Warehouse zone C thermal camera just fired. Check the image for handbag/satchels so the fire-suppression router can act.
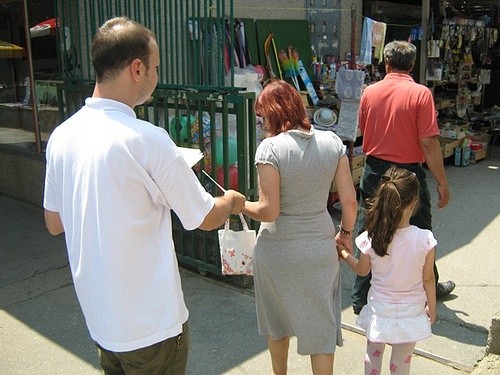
[218,212,256,275]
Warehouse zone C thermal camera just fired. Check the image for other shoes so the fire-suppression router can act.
[437,280,455,297]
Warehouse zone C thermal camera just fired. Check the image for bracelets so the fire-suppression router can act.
[338,224,355,235]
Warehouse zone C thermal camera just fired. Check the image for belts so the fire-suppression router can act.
[367,154,419,166]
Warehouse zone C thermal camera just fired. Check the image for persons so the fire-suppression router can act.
[43,16,246,375]
[351,41,455,315]
[241,79,358,375]
[335,167,437,375]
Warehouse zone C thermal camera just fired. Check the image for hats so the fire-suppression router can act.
[314,108,337,127]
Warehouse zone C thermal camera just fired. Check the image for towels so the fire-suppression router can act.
[359,16,373,66]
[373,21,383,49]
[374,22,387,64]
